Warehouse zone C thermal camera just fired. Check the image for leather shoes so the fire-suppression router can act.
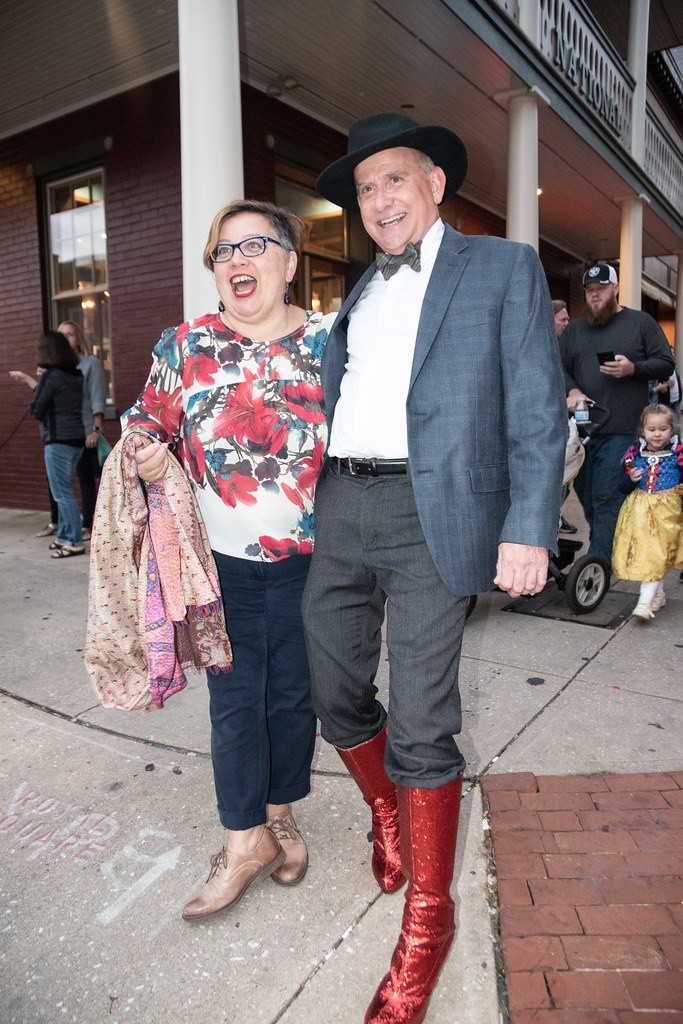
[266,806,309,887]
[181,825,286,922]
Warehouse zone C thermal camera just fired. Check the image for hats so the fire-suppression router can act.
[579,263,617,288]
[315,112,467,215]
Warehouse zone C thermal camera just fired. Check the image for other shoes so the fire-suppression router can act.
[36,525,57,537]
[82,532,90,541]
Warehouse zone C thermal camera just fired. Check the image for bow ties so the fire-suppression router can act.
[376,241,421,281]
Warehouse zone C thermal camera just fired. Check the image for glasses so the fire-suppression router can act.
[210,236,291,263]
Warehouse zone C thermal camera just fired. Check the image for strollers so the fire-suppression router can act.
[465,403,611,619]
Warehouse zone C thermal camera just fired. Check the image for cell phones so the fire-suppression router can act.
[596,350,616,365]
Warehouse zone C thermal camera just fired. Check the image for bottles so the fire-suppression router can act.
[575,399,589,421]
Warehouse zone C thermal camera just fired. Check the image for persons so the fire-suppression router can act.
[612,404,683,621]
[10,322,104,558]
[558,264,675,563]
[552,301,578,532]
[131,202,339,920]
[301,112,567,1024]
[649,345,683,411]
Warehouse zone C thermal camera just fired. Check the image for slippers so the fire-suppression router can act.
[52,548,84,558]
[49,543,61,549]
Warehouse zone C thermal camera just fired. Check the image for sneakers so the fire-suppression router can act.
[631,605,655,620]
[652,591,666,612]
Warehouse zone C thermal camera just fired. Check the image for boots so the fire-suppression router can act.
[334,725,407,895]
[364,773,463,1024]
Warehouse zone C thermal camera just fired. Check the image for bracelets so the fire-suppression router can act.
[93,426,101,431]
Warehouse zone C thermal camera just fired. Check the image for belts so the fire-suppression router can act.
[332,456,408,477]
